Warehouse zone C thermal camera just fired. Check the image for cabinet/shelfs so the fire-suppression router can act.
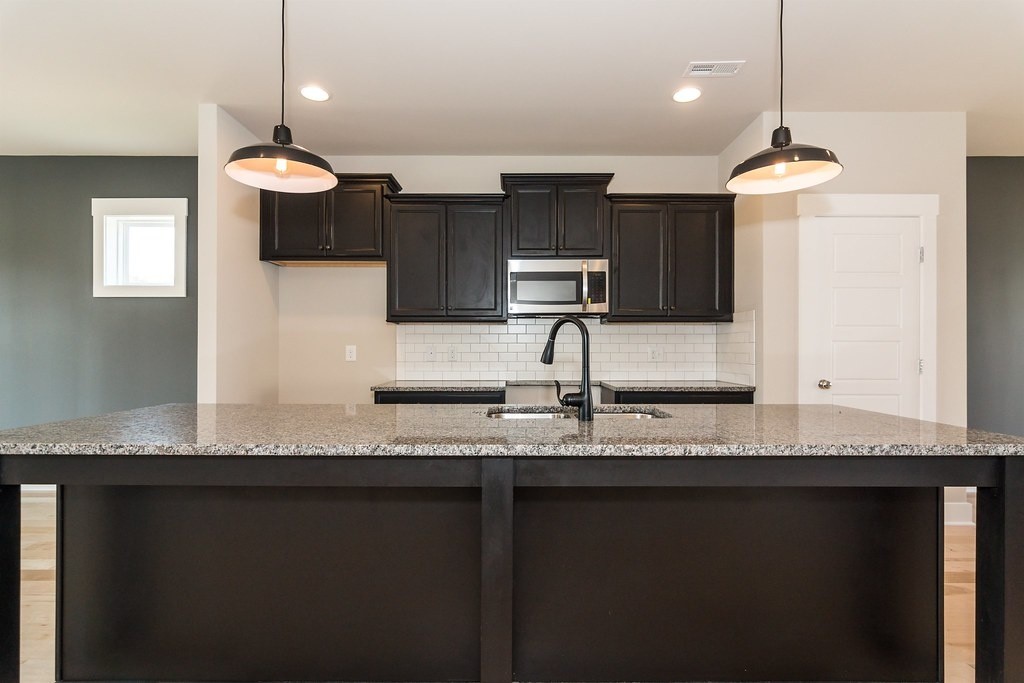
[606,192,737,323]
[500,170,614,258]
[260,171,405,268]
[385,192,508,324]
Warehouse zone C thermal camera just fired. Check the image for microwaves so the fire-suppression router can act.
[507,259,609,316]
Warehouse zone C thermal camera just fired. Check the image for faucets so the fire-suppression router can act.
[540,315,592,420]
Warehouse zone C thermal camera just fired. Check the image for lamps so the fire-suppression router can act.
[724,0,843,195]
[223,0,339,192]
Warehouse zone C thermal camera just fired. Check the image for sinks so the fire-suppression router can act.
[486,406,674,420]
